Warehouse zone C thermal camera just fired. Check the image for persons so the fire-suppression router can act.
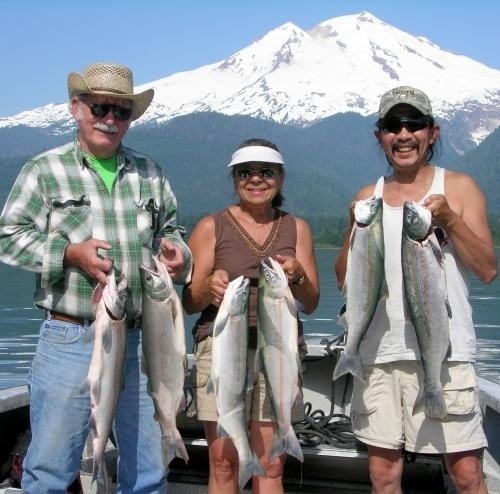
[333,85,497,494]
[182,138,321,493]
[0,62,193,494]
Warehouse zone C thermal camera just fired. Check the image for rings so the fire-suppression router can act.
[438,212,441,217]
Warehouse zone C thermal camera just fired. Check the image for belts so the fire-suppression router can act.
[50,308,144,331]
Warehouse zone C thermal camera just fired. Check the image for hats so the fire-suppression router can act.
[228,145,285,169]
[377,87,434,115]
[66,62,156,120]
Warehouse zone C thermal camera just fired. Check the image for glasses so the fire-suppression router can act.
[235,168,279,179]
[381,116,433,133]
[77,96,132,120]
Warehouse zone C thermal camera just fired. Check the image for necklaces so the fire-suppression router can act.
[245,211,270,237]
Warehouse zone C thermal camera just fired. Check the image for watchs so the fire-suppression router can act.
[292,273,307,288]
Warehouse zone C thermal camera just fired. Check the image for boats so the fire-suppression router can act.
[0,331,498,493]
